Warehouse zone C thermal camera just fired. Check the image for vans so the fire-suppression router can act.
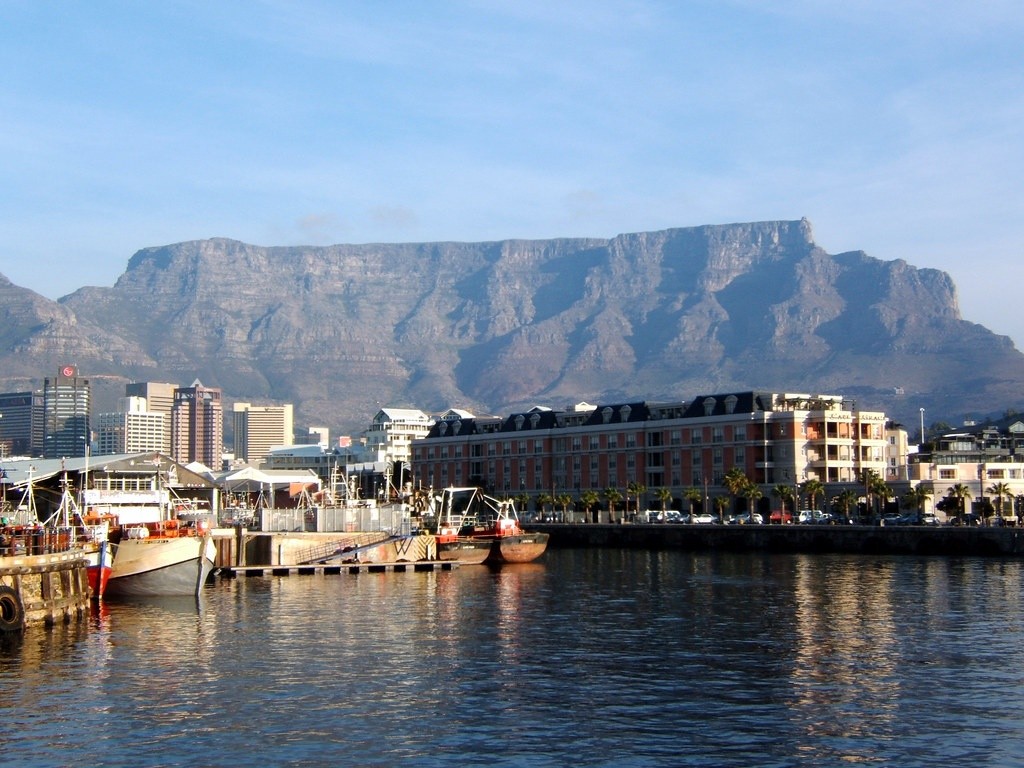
[649,511,680,523]
[792,510,822,524]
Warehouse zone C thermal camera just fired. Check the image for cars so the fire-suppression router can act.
[691,514,718,524]
[951,512,980,526]
[805,513,839,525]
[517,511,564,523]
[730,515,745,523]
[676,514,697,523]
[666,514,679,523]
[902,512,939,526]
[770,509,792,523]
[873,513,903,525]
[742,514,763,524]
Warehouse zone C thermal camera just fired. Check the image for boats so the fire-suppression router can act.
[437,483,494,565]
[44,457,113,601]
[494,494,550,563]
[108,463,218,597]
